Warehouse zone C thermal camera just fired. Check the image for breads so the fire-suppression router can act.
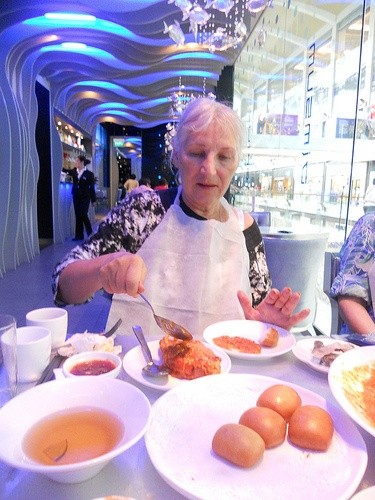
[260,326,278,346]
[238,406,286,449]
[256,384,301,422]
[159,335,221,379]
[288,404,336,450]
[212,423,265,468]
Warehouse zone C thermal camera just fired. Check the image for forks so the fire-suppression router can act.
[102,318,124,338]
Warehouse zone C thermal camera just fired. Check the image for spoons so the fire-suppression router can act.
[132,324,171,376]
[137,291,193,342]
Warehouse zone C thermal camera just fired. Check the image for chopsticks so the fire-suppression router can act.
[36,354,63,385]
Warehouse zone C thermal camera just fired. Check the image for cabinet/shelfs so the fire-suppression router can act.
[53,114,88,184]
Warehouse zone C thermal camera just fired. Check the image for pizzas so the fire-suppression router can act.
[211,336,261,354]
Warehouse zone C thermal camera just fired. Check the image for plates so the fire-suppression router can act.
[293,338,360,372]
[123,339,231,390]
[203,319,295,360]
[145,373,375,500]
[63,351,122,378]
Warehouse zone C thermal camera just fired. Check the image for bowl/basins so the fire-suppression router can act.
[0,375,151,485]
[328,345,375,437]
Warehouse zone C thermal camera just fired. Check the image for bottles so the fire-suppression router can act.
[59,131,85,161]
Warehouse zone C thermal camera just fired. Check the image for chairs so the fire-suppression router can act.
[262,233,329,335]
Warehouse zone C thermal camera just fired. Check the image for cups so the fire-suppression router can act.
[26,307,67,350]
[1,325,52,383]
[0,315,19,408]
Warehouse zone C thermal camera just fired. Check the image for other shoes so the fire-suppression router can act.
[71,237,85,242]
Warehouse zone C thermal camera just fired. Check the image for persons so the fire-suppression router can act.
[154,178,168,190]
[134,178,153,191]
[123,173,139,194]
[52,97,311,332]
[329,209,375,335]
[62,155,98,241]
[230,182,239,205]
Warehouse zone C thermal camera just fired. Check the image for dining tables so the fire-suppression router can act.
[0,332,375,500]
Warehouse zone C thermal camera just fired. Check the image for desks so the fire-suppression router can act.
[257,222,331,240]
